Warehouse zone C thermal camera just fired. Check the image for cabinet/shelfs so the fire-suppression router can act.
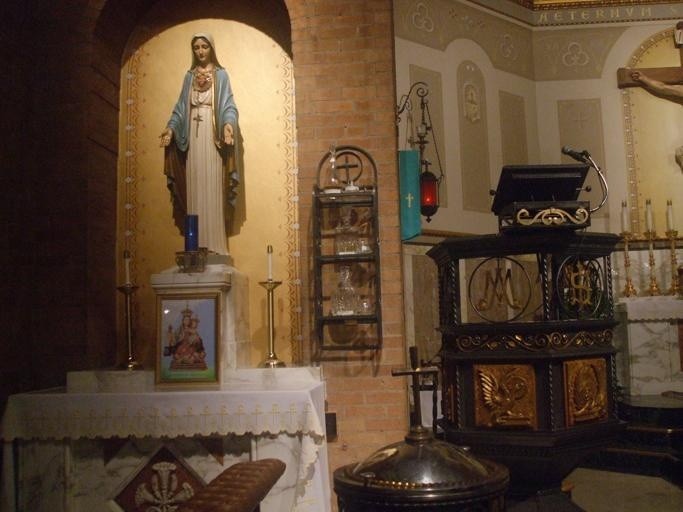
[313,145,383,351]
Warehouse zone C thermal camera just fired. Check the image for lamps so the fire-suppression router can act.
[395,82,446,223]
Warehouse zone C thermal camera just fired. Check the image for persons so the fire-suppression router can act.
[158,32,239,257]
[167,305,207,363]
[631,70,683,172]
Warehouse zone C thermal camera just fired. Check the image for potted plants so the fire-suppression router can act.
[622,201,629,232]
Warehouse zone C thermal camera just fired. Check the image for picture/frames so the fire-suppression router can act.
[154,290,221,390]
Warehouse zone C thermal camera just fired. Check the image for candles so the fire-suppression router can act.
[124,251,130,284]
[646,199,653,231]
[667,200,673,230]
[268,246,272,280]
